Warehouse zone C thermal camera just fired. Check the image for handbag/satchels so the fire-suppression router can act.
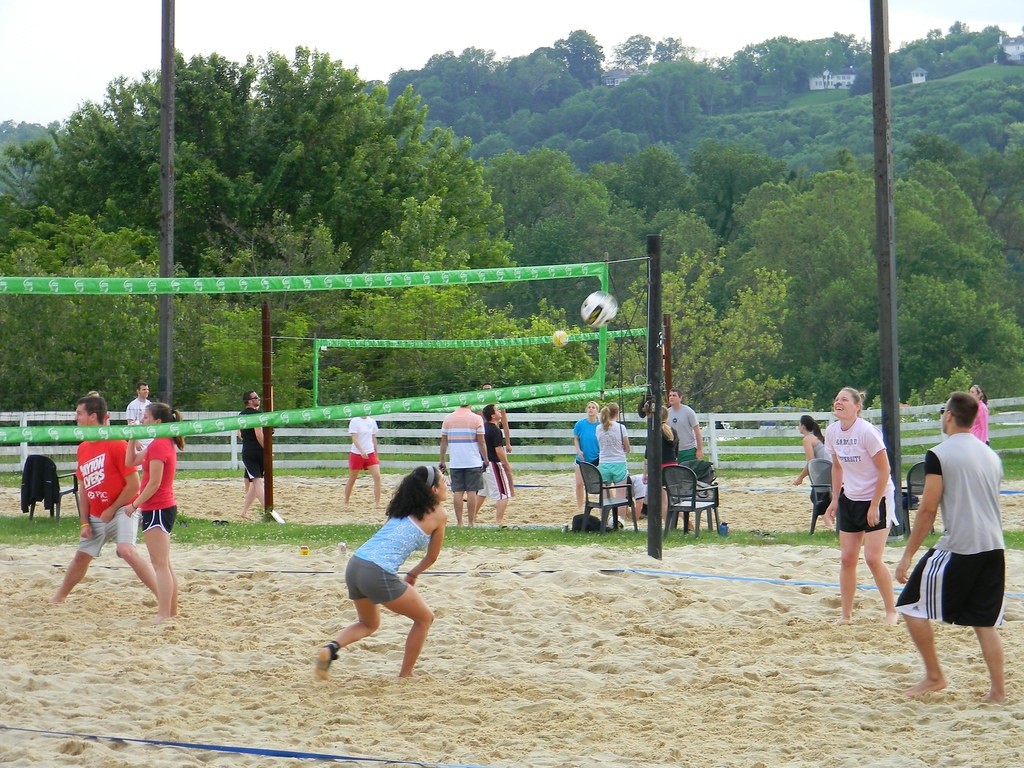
[679,459,717,499]
[572,514,600,532]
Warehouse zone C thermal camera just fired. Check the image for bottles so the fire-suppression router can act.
[337,541,347,554]
[301,546,309,555]
[720,522,728,536]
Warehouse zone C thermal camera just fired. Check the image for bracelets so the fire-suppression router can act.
[80,524,90,527]
[406,572,417,579]
[132,502,137,510]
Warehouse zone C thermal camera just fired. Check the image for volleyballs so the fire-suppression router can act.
[581,291,618,329]
[553,331,568,348]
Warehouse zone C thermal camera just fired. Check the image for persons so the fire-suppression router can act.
[236,389,274,519]
[644,404,695,532]
[343,399,387,509]
[626,473,649,523]
[792,415,844,531]
[474,404,512,525]
[469,384,515,497]
[316,465,448,682]
[573,401,602,518]
[124,402,185,619]
[664,386,704,465]
[971,385,988,446]
[438,405,489,529]
[46,390,159,606]
[824,387,898,627]
[596,402,631,523]
[893,392,1006,706]
[125,382,155,471]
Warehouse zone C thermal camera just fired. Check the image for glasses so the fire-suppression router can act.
[251,395,258,399]
[940,407,950,414]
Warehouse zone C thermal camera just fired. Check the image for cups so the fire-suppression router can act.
[561,524,568,532]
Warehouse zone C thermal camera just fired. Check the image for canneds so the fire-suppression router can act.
[338,542,346,554]
[562,523,568,533]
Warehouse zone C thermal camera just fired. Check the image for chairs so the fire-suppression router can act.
[901,462,935,534]
[807,458,844,534]
[662,464,720,538]
[21,455,81,523]
[576,460,638,536]
